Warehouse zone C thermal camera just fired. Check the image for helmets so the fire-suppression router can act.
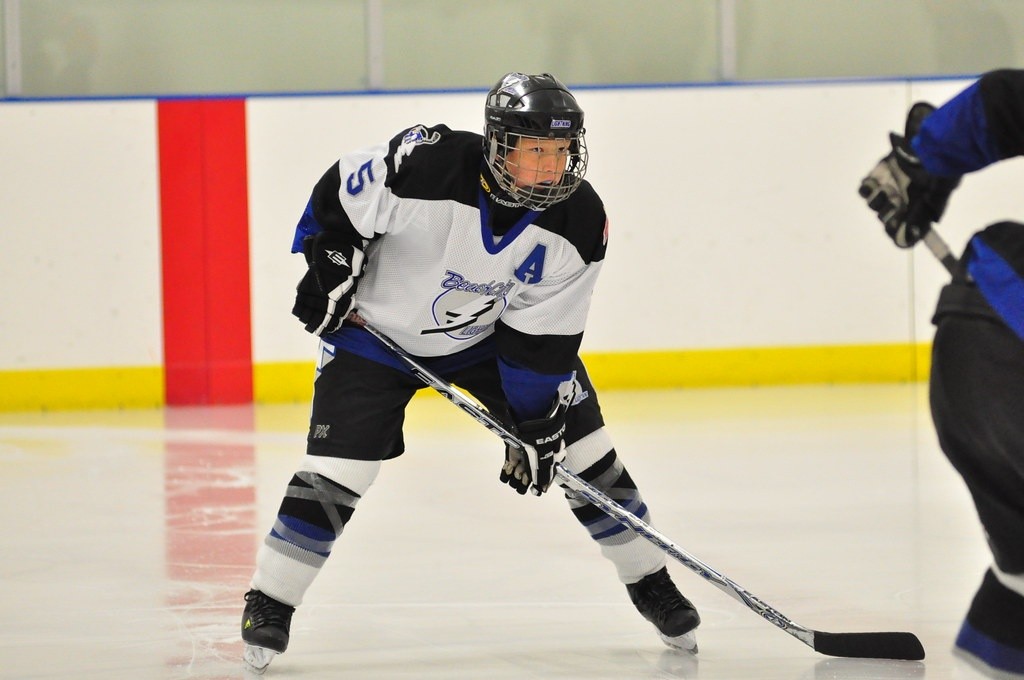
[484,71,585,209]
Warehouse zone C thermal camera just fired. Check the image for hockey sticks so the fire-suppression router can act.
[871,161,960,277]
[346,312,926,659]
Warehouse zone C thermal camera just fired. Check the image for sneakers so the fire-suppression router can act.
[625,566,701,655]
[240,588,296,674]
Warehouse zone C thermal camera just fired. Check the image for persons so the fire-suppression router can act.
[858,69,1024,679]
[241,73,702,672]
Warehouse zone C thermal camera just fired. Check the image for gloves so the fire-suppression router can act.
[859,102,961,249]
[291,230,369,336]
[499,385,566,497]
